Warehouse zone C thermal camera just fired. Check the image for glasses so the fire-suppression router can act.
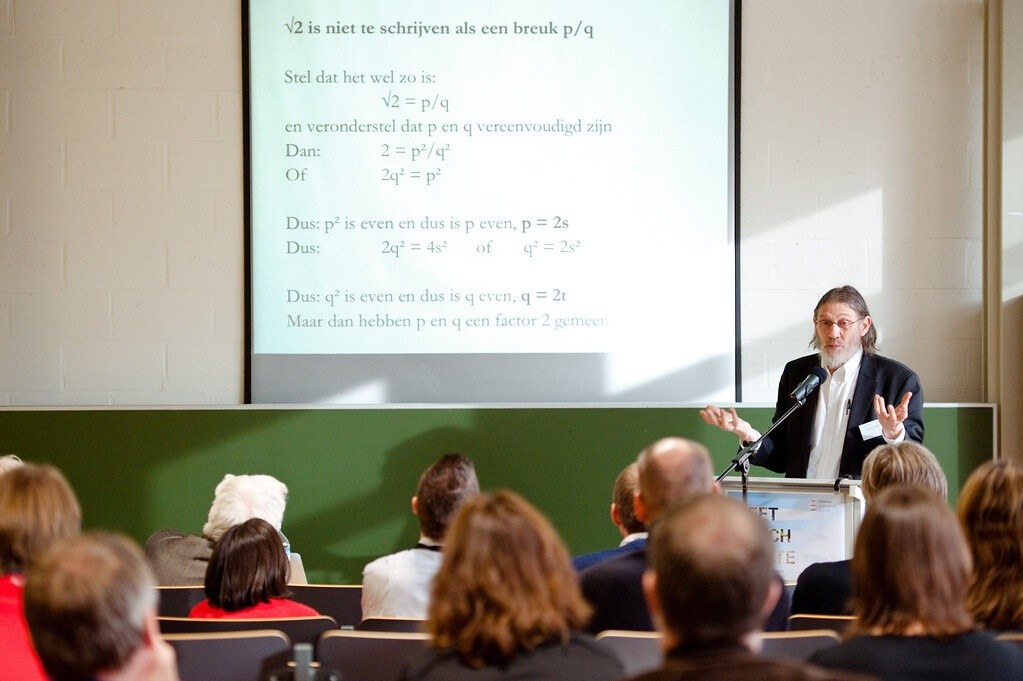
[813,317,865,329]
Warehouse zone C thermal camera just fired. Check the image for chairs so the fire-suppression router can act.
[152,587,859,680]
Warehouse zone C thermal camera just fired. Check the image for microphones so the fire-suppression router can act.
[788,367,827,403]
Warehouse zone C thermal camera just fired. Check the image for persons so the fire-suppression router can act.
[570,463,650,575]
[188,519,321,619]
[142,473,289,587]
[808,485,1023,681]
[580,436,789,638]
[0,464,80,681]
[360,454,480,620]
[958,462,1023,634]
[416,491,628,681]
[699,284,925,480]
[789,442,950,617]
[628,493,872,680]
[24,530,181,681]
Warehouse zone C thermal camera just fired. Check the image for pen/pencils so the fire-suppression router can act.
[846,399,852,415]
